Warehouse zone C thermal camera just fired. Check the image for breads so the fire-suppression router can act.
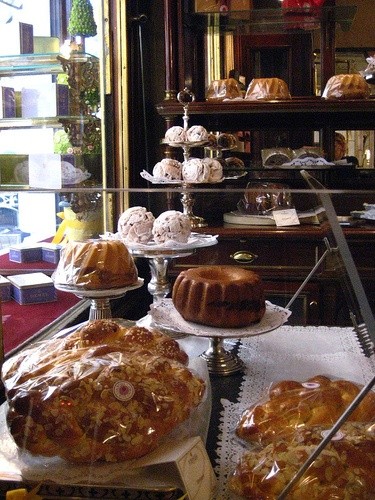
[152,124,223,182]
[172,265,265,329]
[56,240,138,290]
[225,374,375,500]
[205,133,245,177]
[1,319,206,465]
[116,206,192,246]
[332,133,345,160]
[208,77,291,100]
[240,182,292,216]
[321,74,370,100]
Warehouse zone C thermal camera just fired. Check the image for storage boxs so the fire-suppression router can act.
[9,241,63,265]
[0,272,56,306]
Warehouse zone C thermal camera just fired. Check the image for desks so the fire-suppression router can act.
[0,235,91,368]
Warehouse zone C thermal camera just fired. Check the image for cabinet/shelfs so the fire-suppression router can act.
[117,0,375,325]
[0,51,101,239]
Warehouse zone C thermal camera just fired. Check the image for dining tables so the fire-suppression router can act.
[0,318,375,500]
